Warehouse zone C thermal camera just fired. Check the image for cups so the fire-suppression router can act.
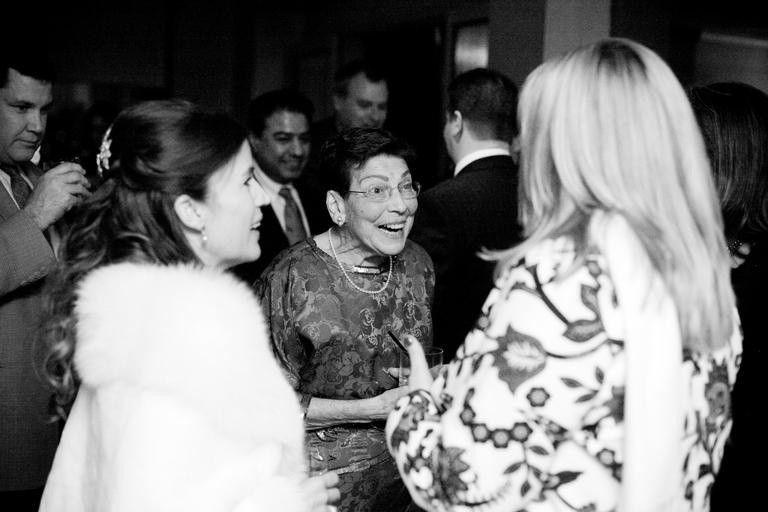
[43,155,83,200]
[399,346,443,387]
[307,445,330,476]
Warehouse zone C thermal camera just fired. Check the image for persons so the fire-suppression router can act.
[704,356,732,421]
[249,129,435,512]
[383,36,743,512]
[226,87,333,288]
[409,68,520,364]
[31,96,339,512]
[0,42,93,512]
[686,81,768,512]
[312,56,389,212]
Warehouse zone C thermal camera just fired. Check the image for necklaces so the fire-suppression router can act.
[327,225,396,298]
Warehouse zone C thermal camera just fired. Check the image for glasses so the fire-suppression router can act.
[340,179,422,203]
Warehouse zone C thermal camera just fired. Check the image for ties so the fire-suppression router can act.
[0,162,53,250]
[279,187,307,246]
